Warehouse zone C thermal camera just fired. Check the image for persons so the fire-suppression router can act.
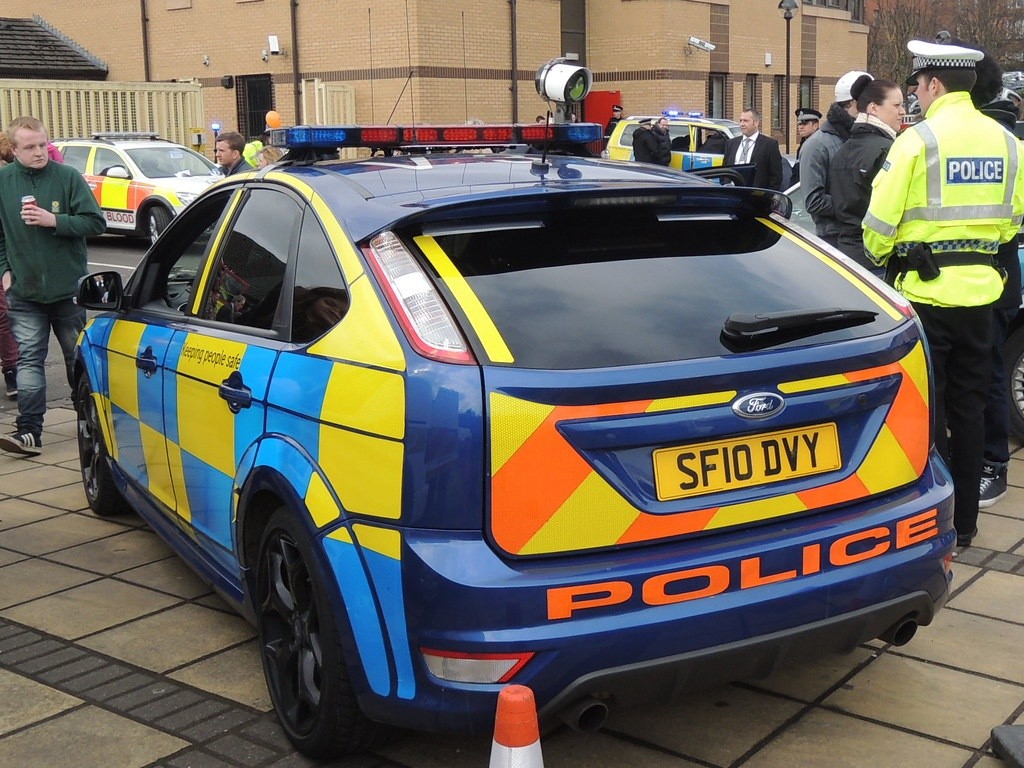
[530,115,550,155]
[720,109,783,193]
[944,41,1024,508]
[204,291,222,318]
[0,117,107,455]
[789,108,822,187]
[829,75,906,282]
[799,71,875,276]
[210,130,256,188]
[256,146,283,173]
[632,118,662,167]
[214,295,246,324]
[861,40,1024,559]
[572,113,579,123]
[650,118,671,169]
[603,104,626,153]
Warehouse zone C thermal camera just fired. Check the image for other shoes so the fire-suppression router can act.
[957,523,978,548]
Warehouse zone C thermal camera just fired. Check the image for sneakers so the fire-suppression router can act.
[0,422,42,455]
[978,465,1008,509]
[1,367,18,396]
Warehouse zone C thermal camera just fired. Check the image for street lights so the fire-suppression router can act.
[778,0,800,154]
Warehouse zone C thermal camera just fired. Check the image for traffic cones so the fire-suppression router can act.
[488,685,545,767]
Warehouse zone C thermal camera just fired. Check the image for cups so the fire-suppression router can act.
[21,196,37,223]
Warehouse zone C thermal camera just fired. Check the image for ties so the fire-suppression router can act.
[738,137,751,163]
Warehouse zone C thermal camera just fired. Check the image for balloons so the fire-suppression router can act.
[265,111,281,129]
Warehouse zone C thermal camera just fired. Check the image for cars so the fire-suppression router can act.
[605,110,741,187]
[73,122,960,768]
[904,70,1024,110]
[52,132,227,246]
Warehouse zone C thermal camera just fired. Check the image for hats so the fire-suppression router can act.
[639,118,652,125]
[794,108,822,121]
[612,105,623,112]
[834,70,875,102]
[897,39,985,85]
